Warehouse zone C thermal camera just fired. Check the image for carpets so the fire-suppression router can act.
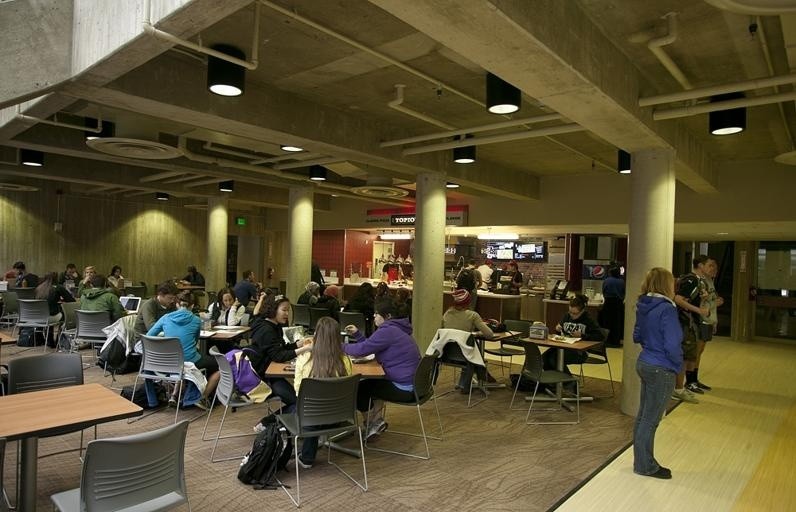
[0,328,691,510]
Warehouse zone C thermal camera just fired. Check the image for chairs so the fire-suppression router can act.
[267,373,368,507]
[201,346,284,463]
[575,328,614,399]
[363,348,446,460]
[3,352,98,509]
[509,338,581,425]
[50,420,190,510]
[0,273,535,427]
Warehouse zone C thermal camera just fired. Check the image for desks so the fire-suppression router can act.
[264,355,386,459]
[1,382,143,512]
[521,335,603,412]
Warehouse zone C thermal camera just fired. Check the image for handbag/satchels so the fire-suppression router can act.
[18,328,44,346]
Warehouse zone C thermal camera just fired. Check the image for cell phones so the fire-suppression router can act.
[284,366,296,370]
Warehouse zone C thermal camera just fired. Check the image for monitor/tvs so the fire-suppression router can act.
[486,241,514,261]
[513,241,545,261]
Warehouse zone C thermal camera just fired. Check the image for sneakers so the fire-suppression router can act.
[297,452,312,468]
[194,399,210,410]
[671,381,711,403]
[169,396,177,406]
[367,419,387,441]
[479,372,497,383]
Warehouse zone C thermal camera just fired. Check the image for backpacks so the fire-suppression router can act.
[456,268,475,290]
[225,348,272,404]
[238,425,292,490]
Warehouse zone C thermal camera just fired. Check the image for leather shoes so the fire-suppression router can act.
[650,467,671,478]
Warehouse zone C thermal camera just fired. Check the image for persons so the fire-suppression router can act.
[670,254,714,404]
[597,264,626,335]
[633,266,685,480]
[695,258,725,391]
[541,294,605,397]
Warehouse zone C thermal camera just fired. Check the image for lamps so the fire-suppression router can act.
[446,182,460,189]
[219,179,235,193]
[309,164,326,181]
[22,150,44,167]
[85,118,107,140]
[206,43,247,97]
[280,145,303,151]
[375,227,415,267]
[485,72,522,115]
[710,92,749,135]
[453,134,476,164]
[156,192,169,201]
[618,147,631,174]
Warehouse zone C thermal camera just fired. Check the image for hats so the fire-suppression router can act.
[452,289,472,305]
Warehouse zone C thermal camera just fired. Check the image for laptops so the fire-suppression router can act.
[119,297,142,314]
